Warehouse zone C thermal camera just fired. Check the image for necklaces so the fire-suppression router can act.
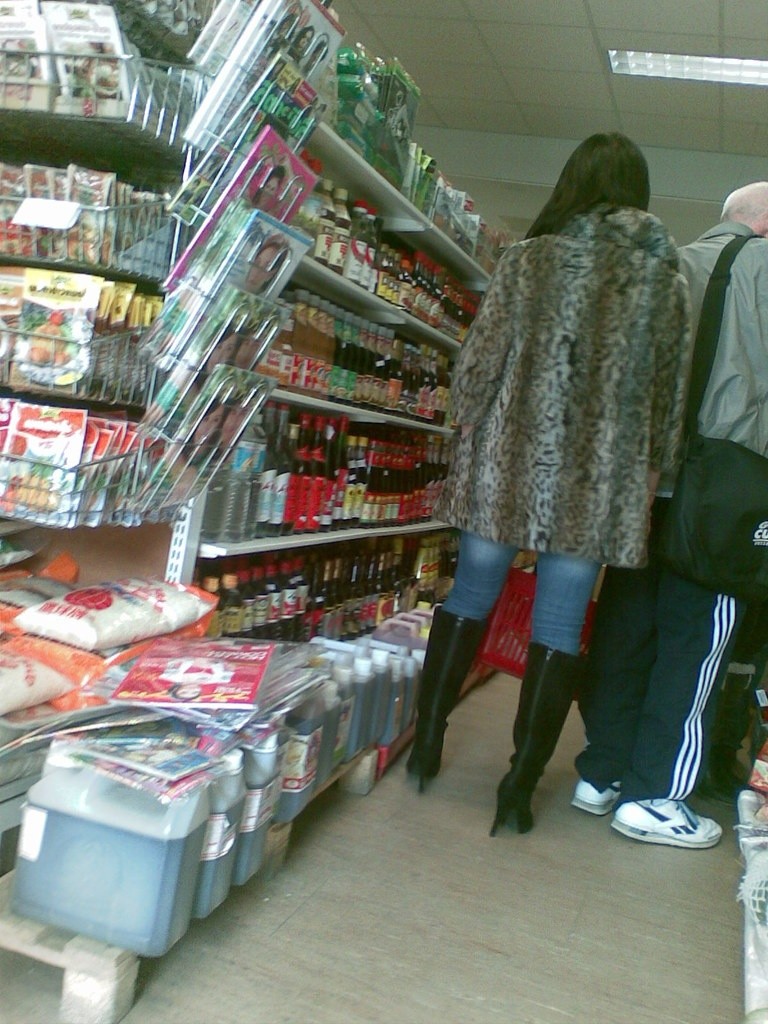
[252,166,286,214]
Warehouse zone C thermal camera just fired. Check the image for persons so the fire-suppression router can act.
[574,181,768,850]
[210,398,259,472]
[408,133,692,838]
[303,41,329,75]
[182,397,224,480]
[244,233,289,295]
[269,14,296,57]
[234,321,266,377]
[195,186,209,208]
[158,657,236,700]
[171,178,199,215]
[193,320,243,388]
[287,26,315,63]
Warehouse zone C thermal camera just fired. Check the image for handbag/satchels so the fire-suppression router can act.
[648,435,768,608]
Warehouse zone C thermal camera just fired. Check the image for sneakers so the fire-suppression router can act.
[571,778,622,816]
[610,799,723,849]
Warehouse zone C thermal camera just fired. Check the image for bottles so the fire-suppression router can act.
[293,150,480,345]
[199,402,449,541]
[12,602,442,958]
[194,533,463,644]
[259,283,456,428]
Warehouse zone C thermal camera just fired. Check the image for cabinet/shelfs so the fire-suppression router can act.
[0,0,492,835]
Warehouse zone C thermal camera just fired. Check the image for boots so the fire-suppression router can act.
[406,606,488,795]
[488,642,582,838]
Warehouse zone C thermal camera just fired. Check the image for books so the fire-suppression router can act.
[0,1,344,800]
